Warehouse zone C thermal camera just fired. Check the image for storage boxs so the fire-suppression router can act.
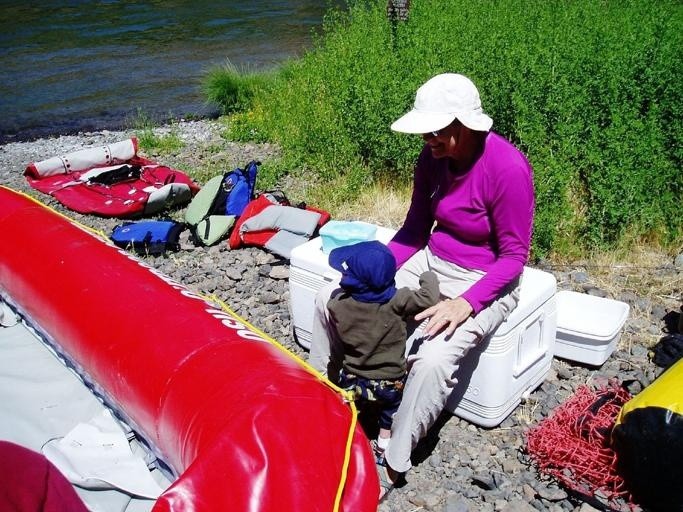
[556,292,627,367]
[291,224,556,427]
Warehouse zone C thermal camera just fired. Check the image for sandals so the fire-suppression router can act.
[375,457,405,504]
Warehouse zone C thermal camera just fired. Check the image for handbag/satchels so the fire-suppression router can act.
[208,160,261,216]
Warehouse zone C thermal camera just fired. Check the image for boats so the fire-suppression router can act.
[0,183,385,512]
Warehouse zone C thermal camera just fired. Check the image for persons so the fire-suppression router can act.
[322,238,440,467]
[303,69,536,505]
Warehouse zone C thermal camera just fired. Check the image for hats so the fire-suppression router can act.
[329,240,397,303]
[390,73,494,134]
